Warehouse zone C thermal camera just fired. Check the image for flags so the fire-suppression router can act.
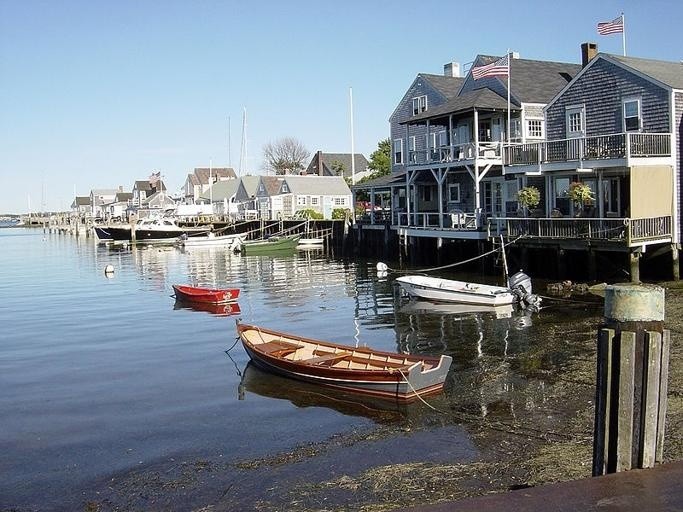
[472,57,510,78]
[596,15,624,34]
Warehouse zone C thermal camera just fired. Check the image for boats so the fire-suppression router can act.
[174,232,248,246]
[234,317,452,402]
[395,273,546,313]
[170,284,240,303]
[172,298,241,318]
[236,359,412,427]
[231,232,301,252]
[396,297,537,331]
[90,219,210,240]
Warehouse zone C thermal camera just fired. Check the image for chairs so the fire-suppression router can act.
[479,143,499,157]
[585,143,609,159]
[620,138,647,157]
[449,208,467,227]
